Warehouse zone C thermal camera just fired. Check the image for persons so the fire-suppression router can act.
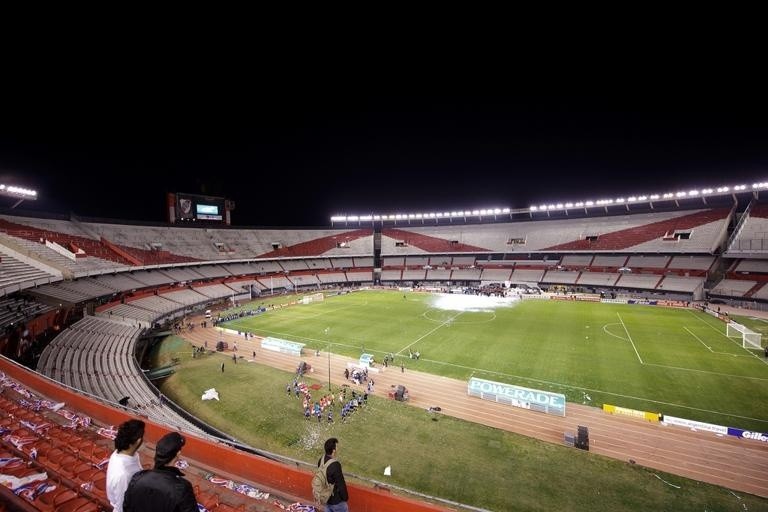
[316,436,350,511]
[285,366,376,426]
[105,417,146,512]
[399,359,405,373]
[120,431,202,512]
[172,306,264,372]
[381,351,395,369]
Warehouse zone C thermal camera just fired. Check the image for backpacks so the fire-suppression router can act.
[310,453,338,505]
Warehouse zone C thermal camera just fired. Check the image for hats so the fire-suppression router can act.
[154,431,186,458]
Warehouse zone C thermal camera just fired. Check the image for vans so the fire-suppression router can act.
[205,310,211,318]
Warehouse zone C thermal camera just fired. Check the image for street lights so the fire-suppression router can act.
[324,326,331,391]
